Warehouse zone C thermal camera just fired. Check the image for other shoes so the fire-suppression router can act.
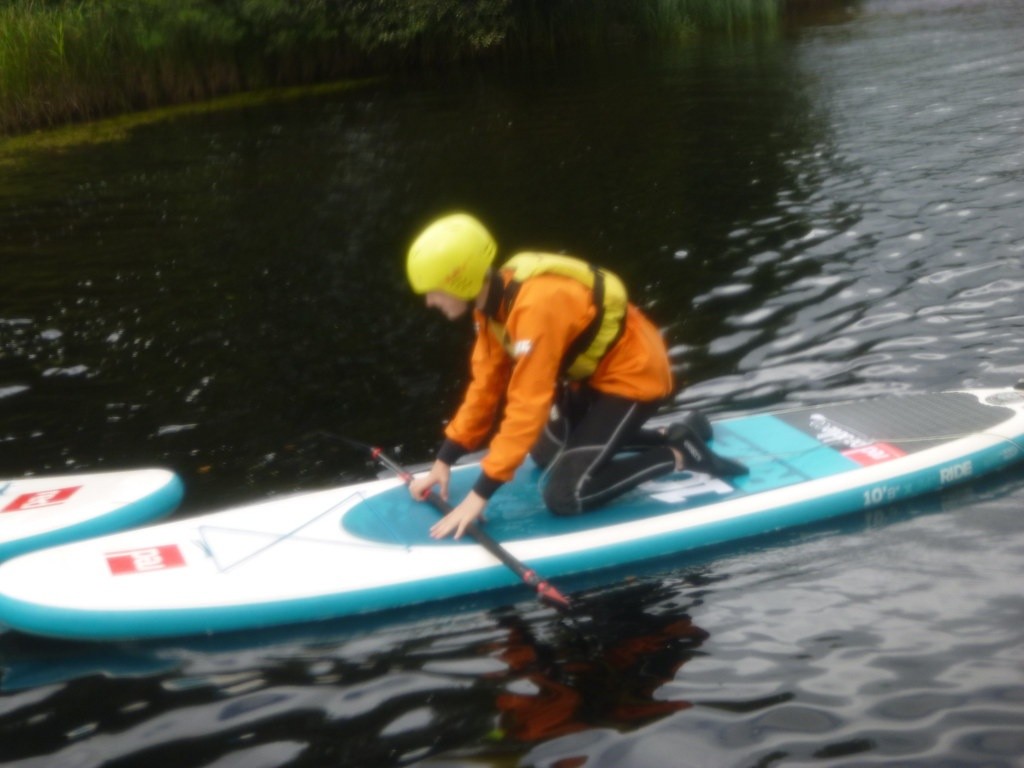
[665,422,748,476]
[684,408,713,440]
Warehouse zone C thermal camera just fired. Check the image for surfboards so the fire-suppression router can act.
[0,463,187,558]
[0,381,1022,646]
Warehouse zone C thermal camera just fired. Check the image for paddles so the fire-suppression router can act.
[364,440,579,613]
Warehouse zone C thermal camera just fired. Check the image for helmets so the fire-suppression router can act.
[406,212,497,300]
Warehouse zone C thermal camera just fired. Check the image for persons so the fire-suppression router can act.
[402,206,748,544]
